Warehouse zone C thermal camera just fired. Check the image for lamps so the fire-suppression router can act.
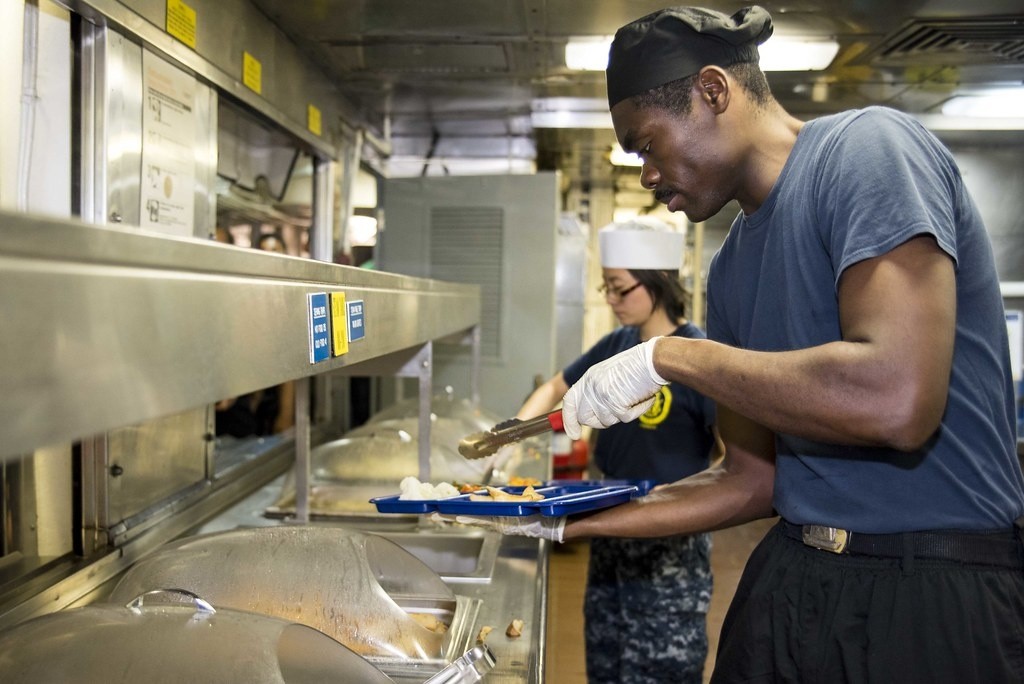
[562,33,843,71]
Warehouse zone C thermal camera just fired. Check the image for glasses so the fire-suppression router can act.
[598,281,643,301]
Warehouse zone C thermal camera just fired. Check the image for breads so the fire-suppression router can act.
[406,612,449,633]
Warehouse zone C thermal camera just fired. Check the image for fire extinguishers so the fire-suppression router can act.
[552,428,589,481]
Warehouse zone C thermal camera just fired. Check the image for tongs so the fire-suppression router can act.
[458,394,657,460]
[423,644,495,684]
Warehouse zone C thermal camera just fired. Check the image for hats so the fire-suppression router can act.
[599,217,683,269]
[606,5,773,111]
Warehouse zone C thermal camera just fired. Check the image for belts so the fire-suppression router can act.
[779,517,1024,568]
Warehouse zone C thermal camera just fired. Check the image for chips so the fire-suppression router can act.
[468,484,545,502]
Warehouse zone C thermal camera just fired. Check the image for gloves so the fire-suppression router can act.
[484,440,523,475]
[562,336,671,441]
[432,510,567,544]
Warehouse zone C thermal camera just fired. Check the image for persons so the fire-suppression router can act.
[491,215,721,684]
[434,2,1024,684]
